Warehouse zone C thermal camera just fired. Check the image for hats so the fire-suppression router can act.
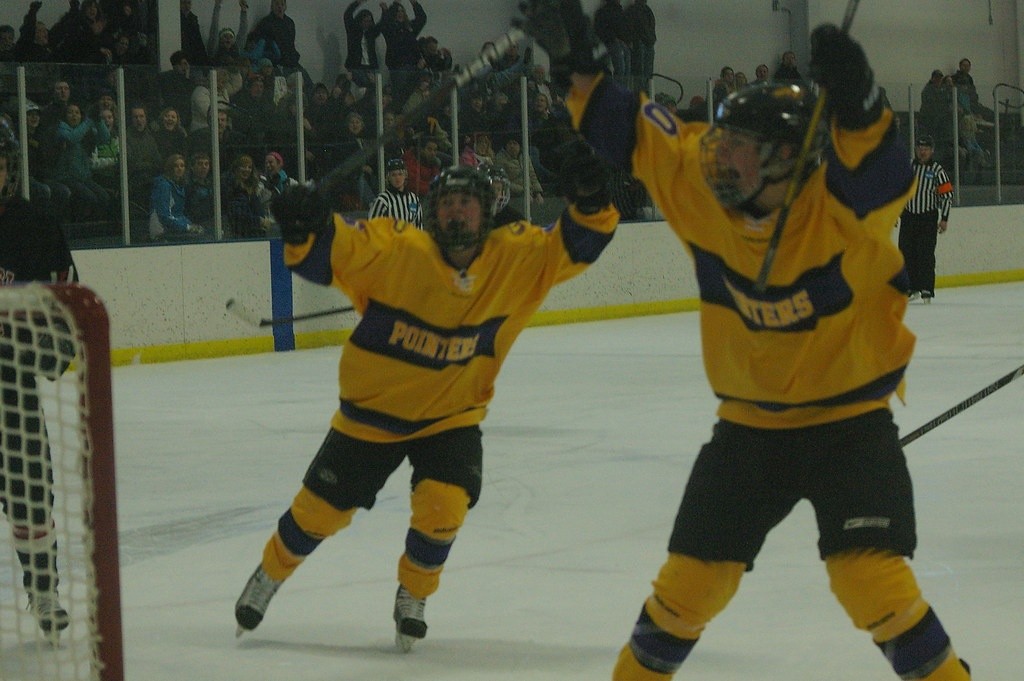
[503,135,520,147]
[25,103,40,115]
[247,73,264,86]
[219,28,235,39]
[931,70,943,77]
[269,151,283,167]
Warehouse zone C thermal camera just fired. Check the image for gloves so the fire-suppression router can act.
[547,138,609,214]
[511,0,597,94]
[808,22,884,132]
[270,184,333,244]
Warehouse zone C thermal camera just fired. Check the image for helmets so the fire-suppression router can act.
[385,158,407,179]
[698,83,828,217]
[423,164,496,250]
[0,113,20,203]
[914,135,935,147]
[479,165,512,214]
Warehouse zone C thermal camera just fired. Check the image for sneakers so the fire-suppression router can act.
[27,587,69,647]
[234,562,279,638]
[393,588,427,653]
[907,290,919,301]
[921,289,932,305]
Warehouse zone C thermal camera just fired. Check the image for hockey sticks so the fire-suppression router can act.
[897,367,1024,450]
[225,296,356,330]
[751,1,860,295]
[321,27,527,194]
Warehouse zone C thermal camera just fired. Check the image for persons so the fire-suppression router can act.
[559,1,680,115]
[510,0,971,680]
[0,1,575,241]
[234,122,618,655]
[899,135,953,305]
[917,58,994,182]
[713,52,799,114]
[0,117,76,637]
